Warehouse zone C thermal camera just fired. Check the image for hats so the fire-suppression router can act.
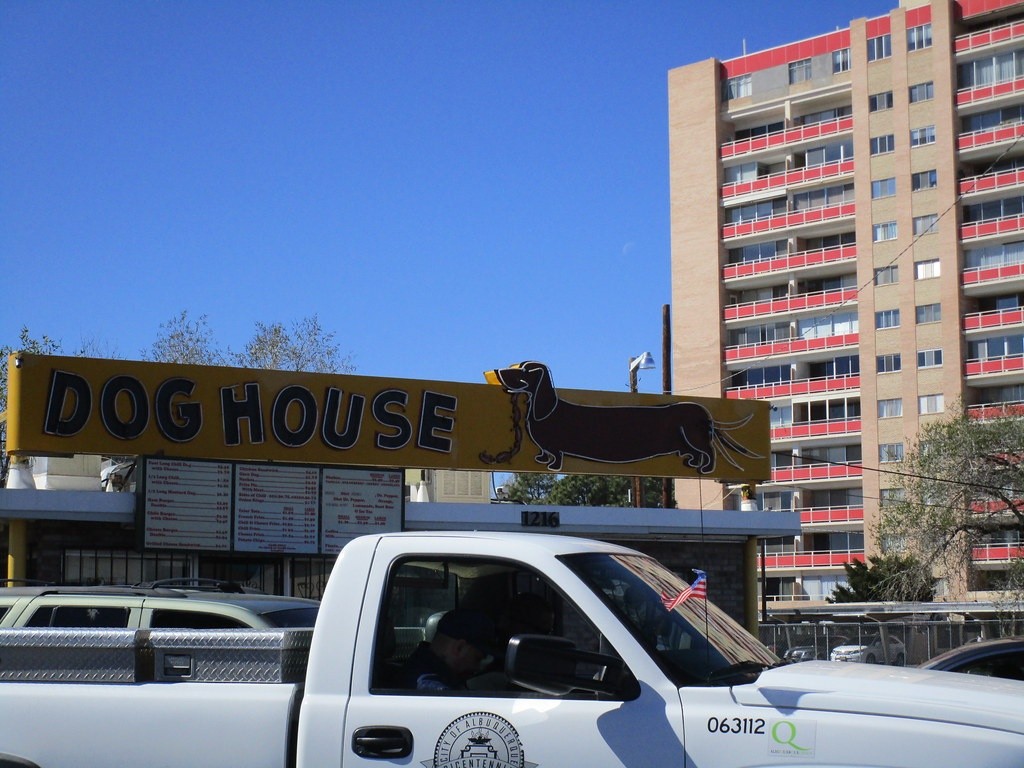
[437,608,506,665]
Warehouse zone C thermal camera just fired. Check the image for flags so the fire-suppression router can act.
[660,568,707,611]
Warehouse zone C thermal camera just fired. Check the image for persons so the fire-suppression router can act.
[398,607,499,689]
[501,594,578,687]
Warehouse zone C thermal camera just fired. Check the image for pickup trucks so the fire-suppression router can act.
[0,531,1024,768]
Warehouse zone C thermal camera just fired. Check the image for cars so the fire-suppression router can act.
[915,635,1024,683]
[830,633,907,666]
[783,634,850,662]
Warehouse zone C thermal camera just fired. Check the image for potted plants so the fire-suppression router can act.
[740,485,758,511]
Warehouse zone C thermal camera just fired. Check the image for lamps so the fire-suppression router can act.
[15,356,24,368]
[768,405,778,411]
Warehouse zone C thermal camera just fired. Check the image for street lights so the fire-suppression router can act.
[626,351,657,508]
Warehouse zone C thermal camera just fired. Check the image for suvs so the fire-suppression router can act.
[0,569,324,629]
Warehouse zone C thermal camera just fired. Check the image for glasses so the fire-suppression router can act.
[536,626,554,635]
